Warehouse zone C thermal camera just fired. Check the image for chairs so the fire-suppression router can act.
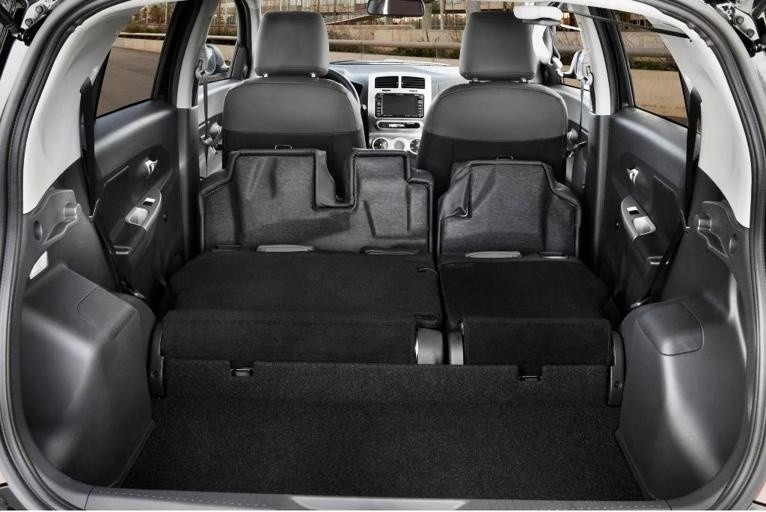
[415,10,567,203]
[221,11,368,199]
